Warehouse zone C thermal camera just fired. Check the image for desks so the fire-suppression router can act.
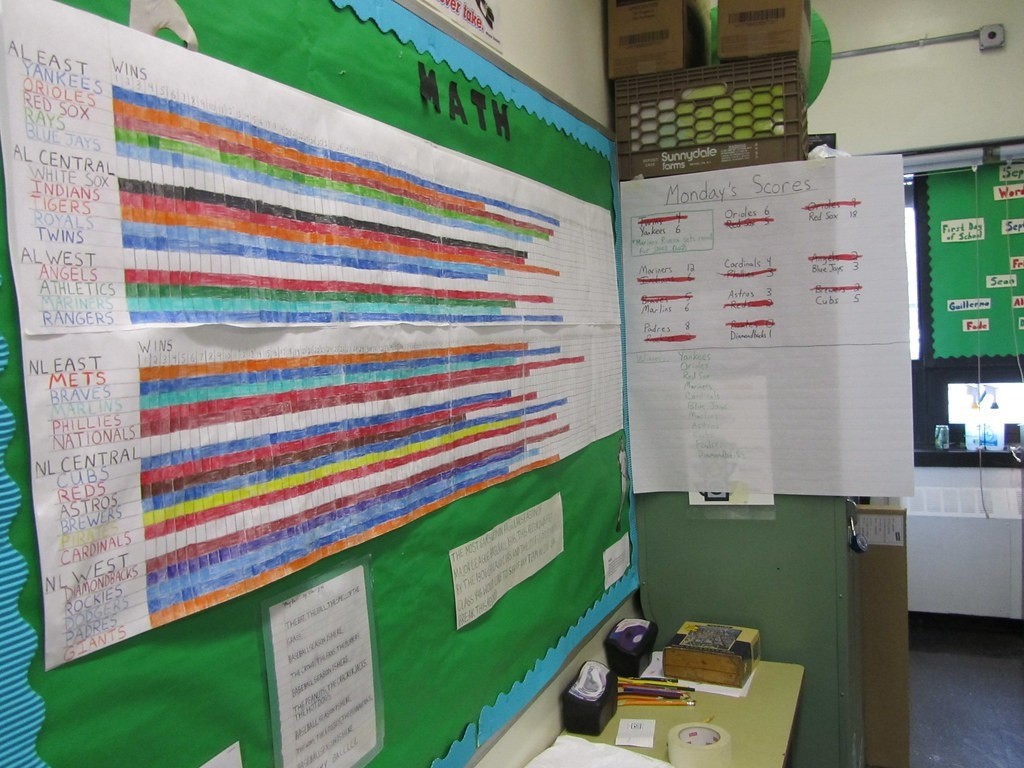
[559,650,805,768]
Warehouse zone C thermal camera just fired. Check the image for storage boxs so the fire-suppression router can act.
[662,621,761,688]
[854,504,909,768]
[607,0,706,80]
[615,54,808,182]
[718,0,812,89]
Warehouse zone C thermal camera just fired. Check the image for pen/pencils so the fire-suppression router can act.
[616,676,696,706]
[666,714,715,747]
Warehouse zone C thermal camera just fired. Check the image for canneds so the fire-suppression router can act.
[935,425,950,450]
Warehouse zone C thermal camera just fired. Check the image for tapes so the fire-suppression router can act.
[668,721,732,768]
